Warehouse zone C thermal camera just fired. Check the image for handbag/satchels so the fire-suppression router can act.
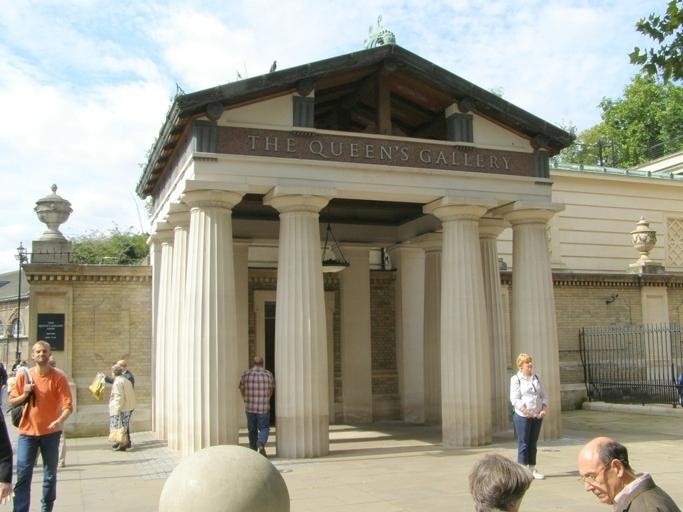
[88,372,107,401]
[108,420,126,444]
[6,366,35,428]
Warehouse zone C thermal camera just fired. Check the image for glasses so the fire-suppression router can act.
[578,457,620,485]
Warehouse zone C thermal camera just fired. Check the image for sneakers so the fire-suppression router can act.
[256,441,266,456]
[528,469,545,479]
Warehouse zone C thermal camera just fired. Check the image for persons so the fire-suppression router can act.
[467,449,534,512]
[108,363,135,451]
[32,354,75,469]
[0,362,15,506]
[575,435,682,512]
[97,359,135,451]
[4,339,75,512]
[7,359,28,377]
[510,351,549,481]
[237,353,276,457]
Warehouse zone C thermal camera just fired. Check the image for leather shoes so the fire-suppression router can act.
[114,446,126,451]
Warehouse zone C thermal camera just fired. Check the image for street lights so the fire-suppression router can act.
[13,241,27,370]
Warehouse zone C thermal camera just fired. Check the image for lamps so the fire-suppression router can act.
[321,198,349,273]
